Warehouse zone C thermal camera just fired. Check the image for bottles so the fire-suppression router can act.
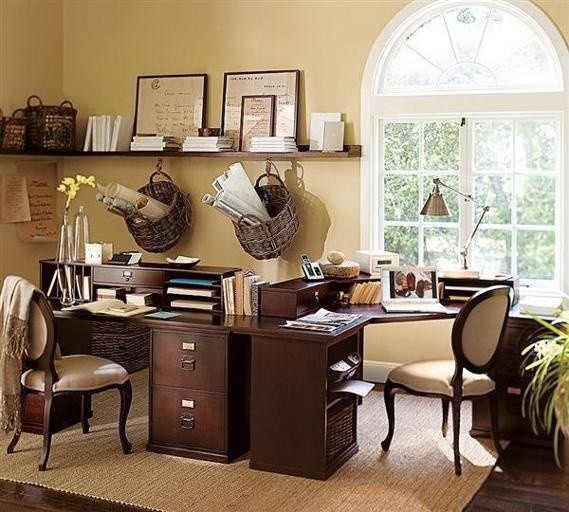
[73,206,90,262]
[55,214,75,263]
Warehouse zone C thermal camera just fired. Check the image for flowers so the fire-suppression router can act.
[57,173,96,223]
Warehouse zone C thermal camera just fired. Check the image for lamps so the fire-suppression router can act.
[419,177,491,270]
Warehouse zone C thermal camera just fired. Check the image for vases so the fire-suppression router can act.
[55,206,91,262]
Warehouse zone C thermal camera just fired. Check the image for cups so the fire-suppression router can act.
[60,288,75,307]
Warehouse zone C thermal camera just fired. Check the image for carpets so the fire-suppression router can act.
[1,367,509,511]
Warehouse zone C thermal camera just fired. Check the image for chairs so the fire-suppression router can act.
[380,286,515,477]
[5,274,132,471]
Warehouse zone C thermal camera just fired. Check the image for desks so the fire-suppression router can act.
[20,292,561,481]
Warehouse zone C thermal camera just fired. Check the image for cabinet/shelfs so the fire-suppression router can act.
[38,257,241,314]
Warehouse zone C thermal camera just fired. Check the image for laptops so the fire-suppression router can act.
[380,265,450,313]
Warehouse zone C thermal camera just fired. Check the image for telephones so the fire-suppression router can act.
[301,253,324,280]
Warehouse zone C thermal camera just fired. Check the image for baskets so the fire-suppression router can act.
[90,318,150,375]
[124,170,192,254]
[232,173,299,261]
[0,109,29,155]
[26,95,77,155]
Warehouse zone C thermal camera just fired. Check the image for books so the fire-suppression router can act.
[167,271,269,316]
[279,307,363,333]
[82,114,300,153]
[350,281,382,304]
[46,268,158,318]
